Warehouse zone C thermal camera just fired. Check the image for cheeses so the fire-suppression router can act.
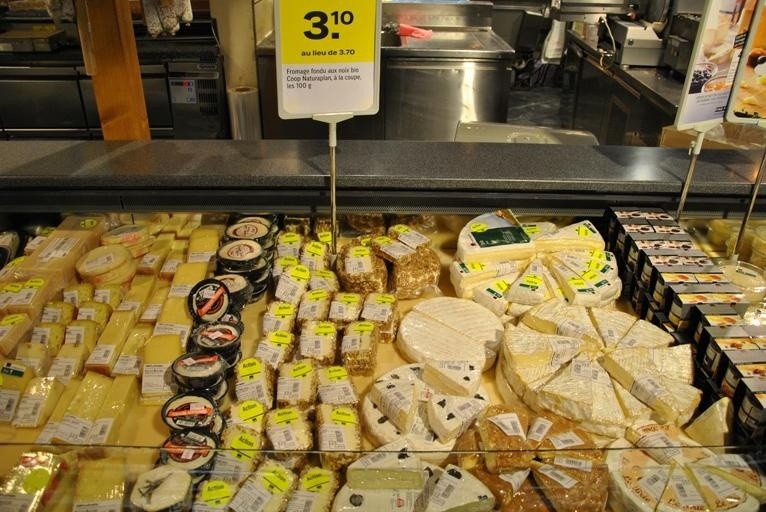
[1,209,766,510]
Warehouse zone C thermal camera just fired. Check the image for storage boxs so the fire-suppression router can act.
[659,122,766,151]
